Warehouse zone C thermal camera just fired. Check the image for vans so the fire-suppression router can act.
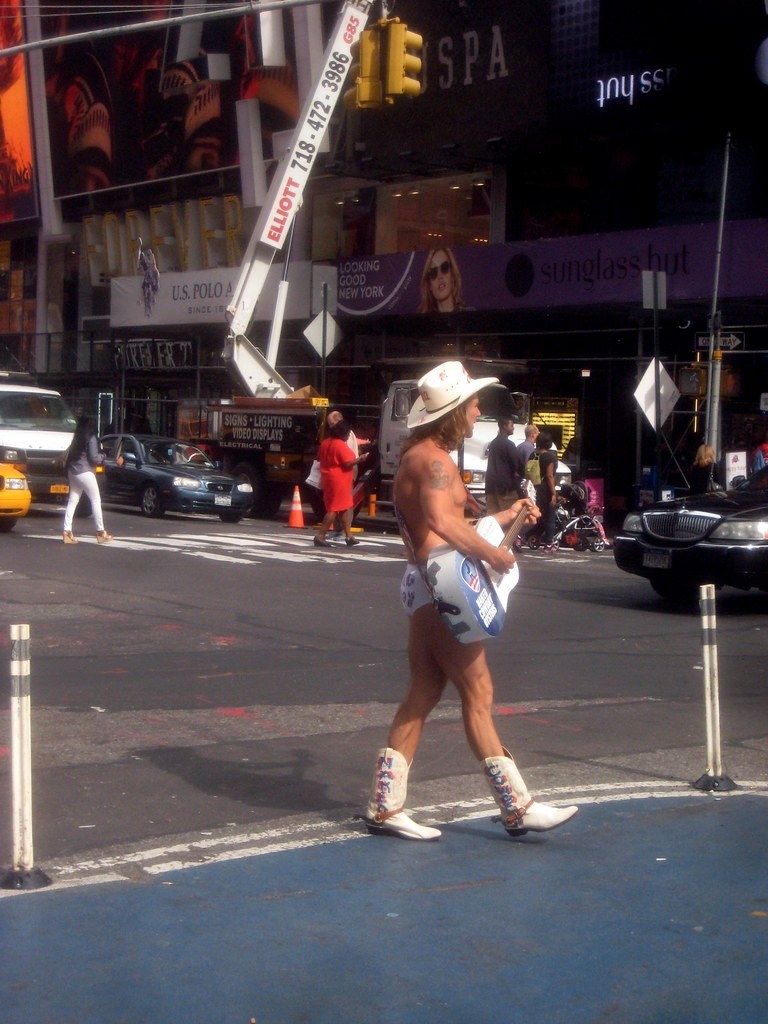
[0,384,105,517]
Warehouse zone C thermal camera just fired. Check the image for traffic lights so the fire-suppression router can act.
[343,30,381,109]
[385,24,423,99]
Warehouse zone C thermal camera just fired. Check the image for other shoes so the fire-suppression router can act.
[602,538,610,545]
[324,532,347,542]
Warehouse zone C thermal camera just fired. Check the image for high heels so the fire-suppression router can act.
[312,537,332,548]
[344,536,360,547]
[62,530,81,545]
[96,529,115,544]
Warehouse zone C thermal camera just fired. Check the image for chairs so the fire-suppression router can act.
[124,439,135,453]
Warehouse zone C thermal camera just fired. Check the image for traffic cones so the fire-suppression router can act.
[283,485,308,529]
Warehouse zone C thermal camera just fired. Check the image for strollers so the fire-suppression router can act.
[528,480,605,553]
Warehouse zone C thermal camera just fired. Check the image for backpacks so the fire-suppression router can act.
[526,451,552,489]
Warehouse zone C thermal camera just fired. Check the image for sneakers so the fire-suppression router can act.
[543,544,558,553]
[513,536,523,553]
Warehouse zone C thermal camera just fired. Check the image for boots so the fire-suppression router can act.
[365,747,446,841]
[477,746,586,836]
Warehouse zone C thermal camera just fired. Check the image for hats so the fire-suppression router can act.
[404,360,503,427]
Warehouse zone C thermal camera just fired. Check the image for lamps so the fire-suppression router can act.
[409,187,420,194]
[449,180,463,190]
[473,176,485,186]
[392,188,404,196]
[336,195,345,204]
[352,191,360,202]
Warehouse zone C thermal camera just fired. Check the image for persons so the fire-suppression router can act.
[312,419,370,546]
[358,360,581,837]
[37,0,307,197]
[481,416,561,555]
[61,412,113,545]
[417,248,478,313]
[689,444,721,498]
[328,410,359,542]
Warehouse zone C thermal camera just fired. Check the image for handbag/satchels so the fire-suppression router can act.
[706,472,723,494]
[752,450,766,474]
[305,459,324,489]
[59,445,76,469]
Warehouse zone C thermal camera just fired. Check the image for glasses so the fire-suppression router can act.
[428,260,454,279]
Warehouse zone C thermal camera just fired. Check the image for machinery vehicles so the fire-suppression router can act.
[219,0,558,521]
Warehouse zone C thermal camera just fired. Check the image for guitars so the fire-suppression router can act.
[426,477,538,645]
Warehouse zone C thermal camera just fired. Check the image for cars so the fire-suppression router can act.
[0,463,32,532]
[100,434,254,523]
[613,465,768,603]
